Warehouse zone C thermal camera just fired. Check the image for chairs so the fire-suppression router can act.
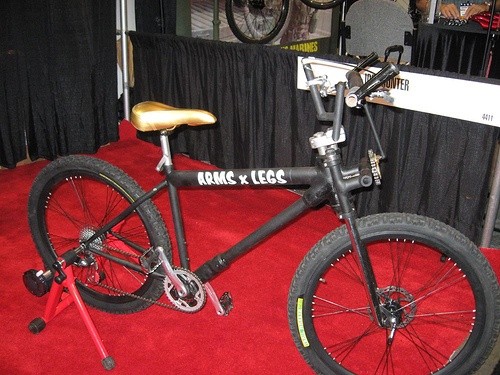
[339,0,413,63]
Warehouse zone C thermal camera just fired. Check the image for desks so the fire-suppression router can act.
[418,22,500,75]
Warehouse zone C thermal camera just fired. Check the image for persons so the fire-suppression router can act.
[415,0,500,21]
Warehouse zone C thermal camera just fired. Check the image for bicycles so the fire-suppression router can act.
[22,51,500,375]
[225,0,345,44]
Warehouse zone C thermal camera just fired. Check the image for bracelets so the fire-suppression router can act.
[485,1,492,11]
